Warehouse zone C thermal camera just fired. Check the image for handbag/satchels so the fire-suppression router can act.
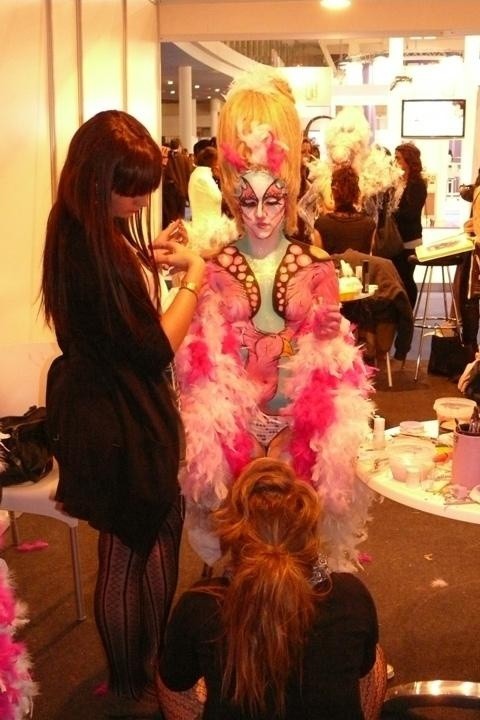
[0,406,56,487]
[372,219,405,258]
[429,336,477,378]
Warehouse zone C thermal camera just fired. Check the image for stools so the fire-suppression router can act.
[0,456,87,622]
[402,255,462,381]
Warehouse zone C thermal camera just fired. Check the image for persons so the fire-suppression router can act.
[170,60,379,581]
[308,166,375,257]
[384,133,426,308]
[295,101,406,231]
[160,134,228,226]
[297,137,310,241]
[155,456,389,720]
[308,142,321,161]
[42,110,207,720]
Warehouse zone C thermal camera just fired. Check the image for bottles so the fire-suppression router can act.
[355,259,370,293]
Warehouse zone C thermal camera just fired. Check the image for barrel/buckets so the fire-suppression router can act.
[432,398,477,436]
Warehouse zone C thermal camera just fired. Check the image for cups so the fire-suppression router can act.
[452,423,480,487]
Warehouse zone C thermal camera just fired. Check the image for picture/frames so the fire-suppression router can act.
[402,99,466,137]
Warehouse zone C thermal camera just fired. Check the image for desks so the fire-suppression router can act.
[356,419,480,524]
[339,284,378,303]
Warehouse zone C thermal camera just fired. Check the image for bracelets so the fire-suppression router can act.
[177,278,205,312]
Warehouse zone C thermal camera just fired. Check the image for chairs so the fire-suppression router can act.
[329,248,392,387]
